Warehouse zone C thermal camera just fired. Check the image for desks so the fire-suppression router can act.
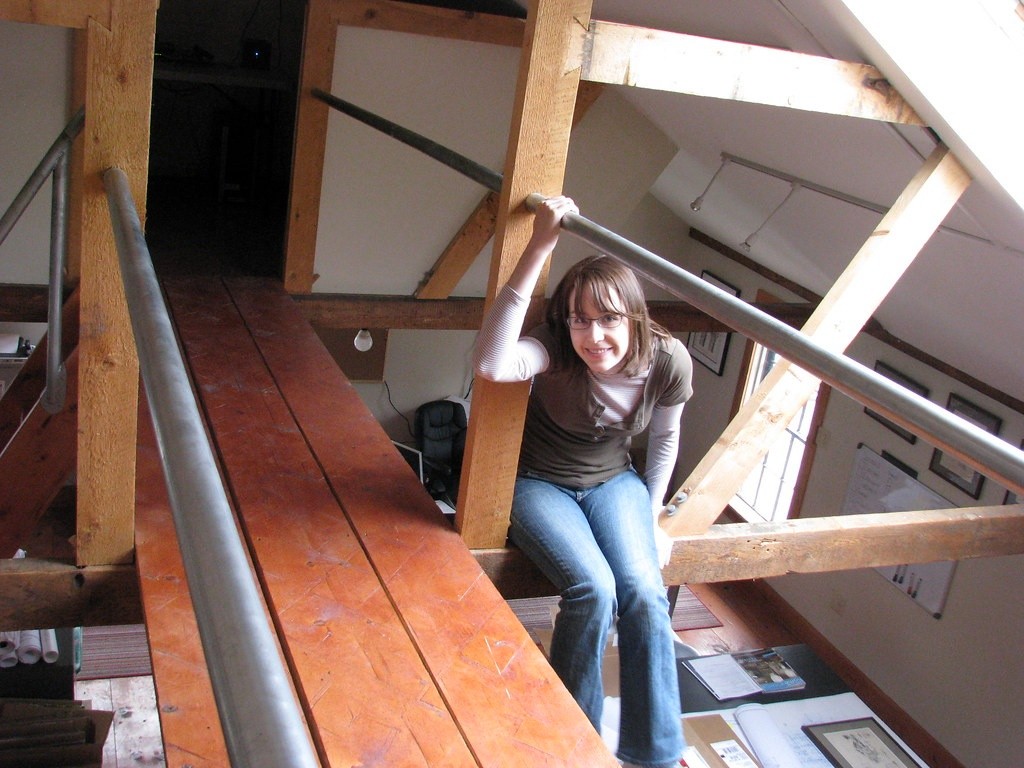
[677,642,931,768]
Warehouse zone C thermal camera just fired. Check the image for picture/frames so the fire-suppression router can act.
[686,269,741,377]
[928,392,1003,500]
[1002,439,1024,506]
[863,360,930,446]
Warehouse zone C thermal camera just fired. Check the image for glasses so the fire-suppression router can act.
[567,313,627,330]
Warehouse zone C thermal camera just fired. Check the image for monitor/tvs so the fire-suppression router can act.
[391,440,424,485]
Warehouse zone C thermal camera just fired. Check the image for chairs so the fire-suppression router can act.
[413,400,467,510]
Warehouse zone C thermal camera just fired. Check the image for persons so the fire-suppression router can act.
[472,194,693,768]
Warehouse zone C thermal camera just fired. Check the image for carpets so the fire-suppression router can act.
[72,584,724,681]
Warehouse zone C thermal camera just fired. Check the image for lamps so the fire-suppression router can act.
[740,181,802,253]
[689,157,731,212]
[354,329,373,352]
